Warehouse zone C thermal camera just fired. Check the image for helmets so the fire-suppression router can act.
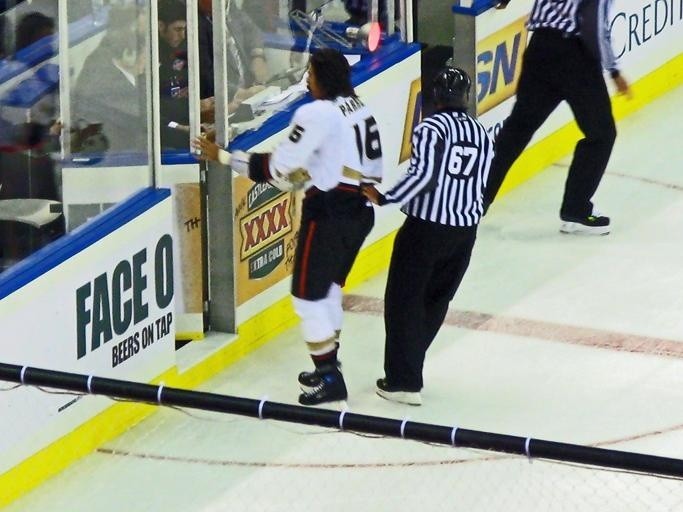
[432,64,472,106]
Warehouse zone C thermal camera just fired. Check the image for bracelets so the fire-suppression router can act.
[378,194,386,204]
[611,70,617,77]
[217,147,230,166]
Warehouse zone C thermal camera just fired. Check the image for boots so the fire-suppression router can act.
[298,341,341,386]
[297,348,348,405]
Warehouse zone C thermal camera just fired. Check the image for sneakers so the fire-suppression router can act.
[560,209,610,227]
[376,375,424,392]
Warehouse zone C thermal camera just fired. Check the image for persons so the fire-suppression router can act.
[0,0,399,170]
[193,49,384,406]
[482,0,634,237]
[360,69,493,405]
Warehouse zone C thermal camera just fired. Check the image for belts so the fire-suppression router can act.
[561,31,575,40]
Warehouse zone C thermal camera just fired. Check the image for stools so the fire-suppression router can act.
[0,197,62,249]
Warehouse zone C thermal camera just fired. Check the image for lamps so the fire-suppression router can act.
[285,3,385,58]
